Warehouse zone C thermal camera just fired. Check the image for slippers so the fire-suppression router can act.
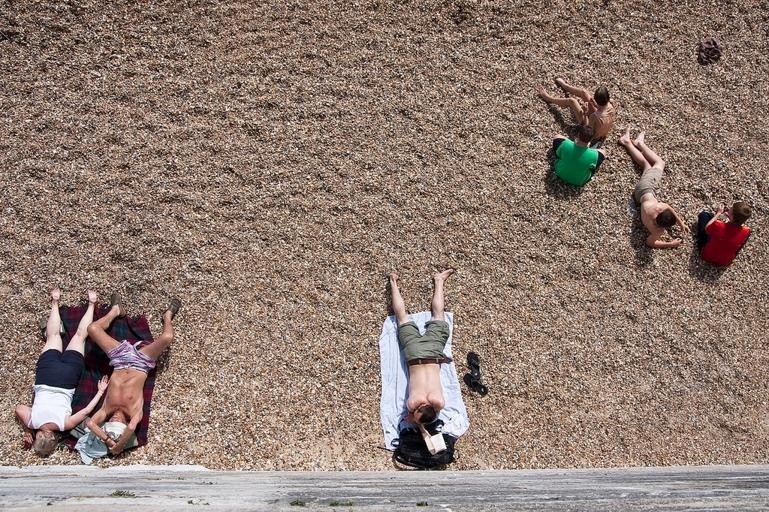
[462,372,489,398]
[466,350,481,381]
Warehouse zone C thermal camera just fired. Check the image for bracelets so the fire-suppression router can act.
[103,436,110,441]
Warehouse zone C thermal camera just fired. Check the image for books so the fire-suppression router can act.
[420,430,448,456]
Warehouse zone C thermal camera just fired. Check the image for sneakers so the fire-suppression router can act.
[110,289,127,318]
[160,295,183,324]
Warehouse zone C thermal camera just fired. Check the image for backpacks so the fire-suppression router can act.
[389,418,461,470]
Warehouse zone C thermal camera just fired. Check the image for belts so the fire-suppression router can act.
[407,355,449,367]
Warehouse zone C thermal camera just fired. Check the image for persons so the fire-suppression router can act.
[385,268,456,433]
[537,76,616,142]
[85,290,182,457]
[16,286,111,458]
[696,200,753,268]
[617,123,692,249]
[550,125,607,187]
